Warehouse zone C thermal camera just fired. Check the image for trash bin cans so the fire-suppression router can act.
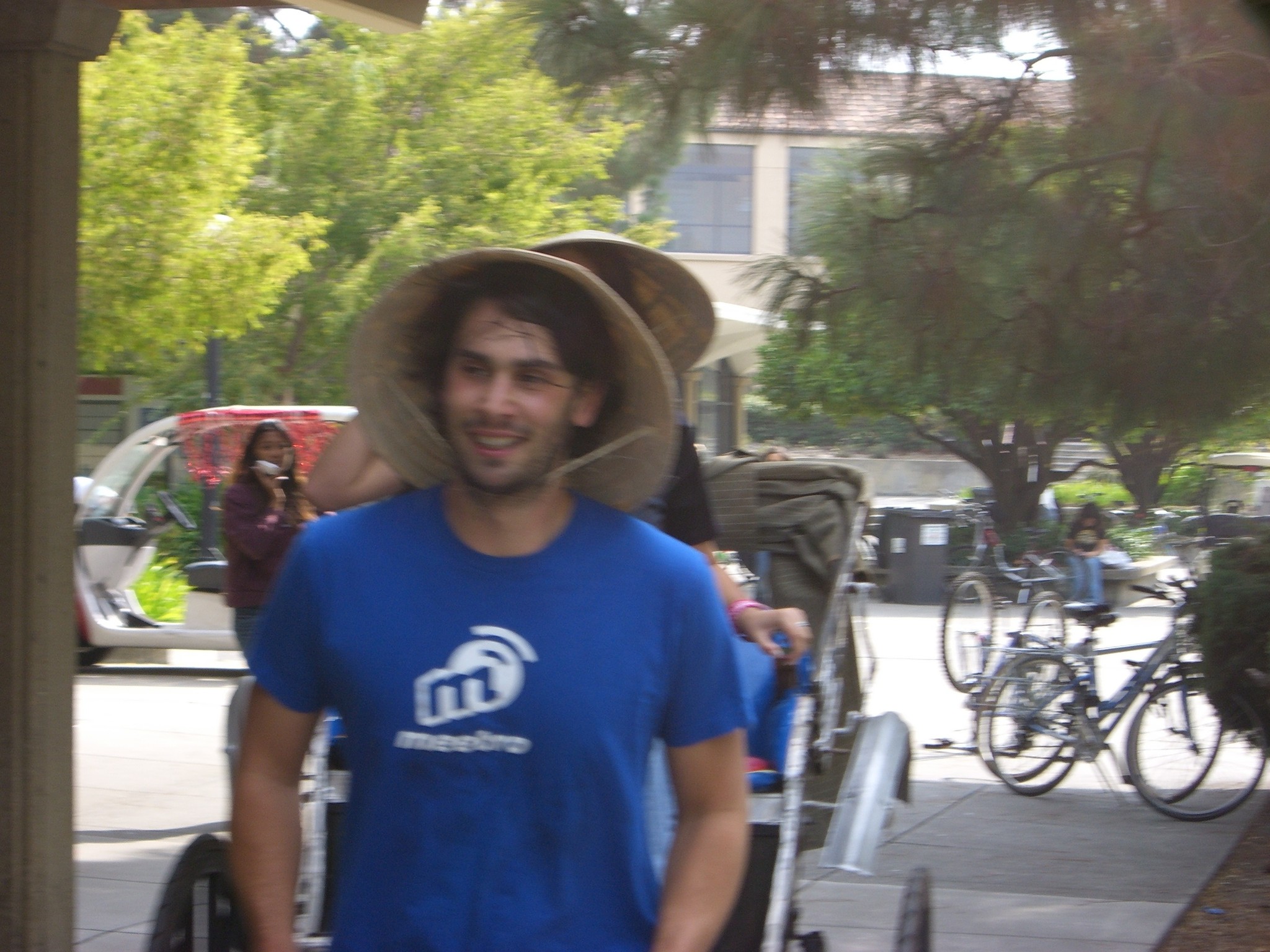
[885,511,951,606]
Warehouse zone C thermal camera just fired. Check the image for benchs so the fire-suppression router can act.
[186,558,231,589]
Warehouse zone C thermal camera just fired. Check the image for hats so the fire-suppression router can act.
[348,244,678,509]
[527,230,715,376]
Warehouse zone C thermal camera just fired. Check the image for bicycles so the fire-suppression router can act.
[924,566,1267,822]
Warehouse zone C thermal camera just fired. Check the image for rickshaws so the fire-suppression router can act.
[144,448,935,952]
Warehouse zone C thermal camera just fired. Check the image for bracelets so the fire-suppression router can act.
[726,598,773,633]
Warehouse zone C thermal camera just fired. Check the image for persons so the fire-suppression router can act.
[308,228,810,904]
[235,248,758,952]
[1063,503,1105,606]
[224,421,324,672]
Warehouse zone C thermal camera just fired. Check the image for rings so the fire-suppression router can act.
[796,621,810,627]
